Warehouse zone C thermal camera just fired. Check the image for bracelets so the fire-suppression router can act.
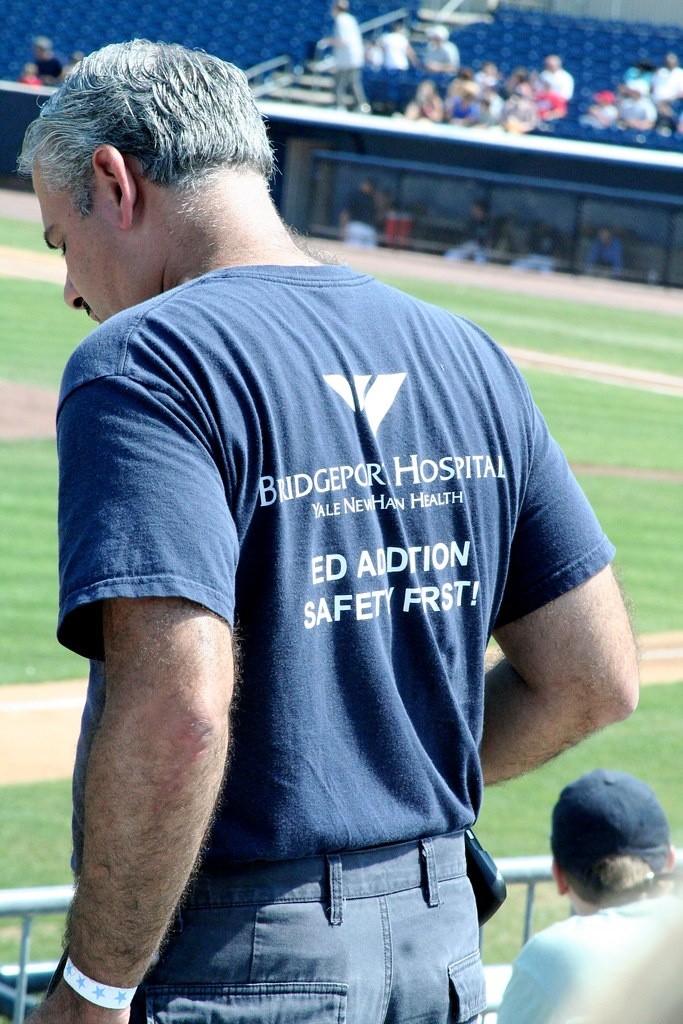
[63,957,138,1009]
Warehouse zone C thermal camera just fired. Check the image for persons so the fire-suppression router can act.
[443,199,497,263]
[586,54,683,131]
[406,79,444,122]
[364,23,461,113]
[586,226,624,280]
[323,0,371,116]
[444,54,574,134]
[495,771,683,1024]
[14,38,639,1024]
[19,36,85,86]
[513,221,564,272]
[337,178,386,247]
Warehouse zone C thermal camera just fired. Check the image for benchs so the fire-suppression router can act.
[412,205,668,287]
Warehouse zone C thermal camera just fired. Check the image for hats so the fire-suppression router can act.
[550,769,672,895]
